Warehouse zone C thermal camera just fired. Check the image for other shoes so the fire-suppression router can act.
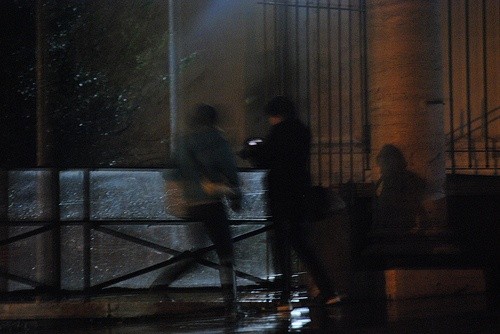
[309,292,340,306]
[226,308,252,325]
[270,301,294,310]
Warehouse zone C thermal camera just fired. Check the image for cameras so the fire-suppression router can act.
[237,137,265,161]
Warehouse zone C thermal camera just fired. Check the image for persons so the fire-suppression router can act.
[238,97,336,312]
[145,104,248,324]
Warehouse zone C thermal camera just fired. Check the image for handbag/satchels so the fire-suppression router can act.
[202,172,237,201]
